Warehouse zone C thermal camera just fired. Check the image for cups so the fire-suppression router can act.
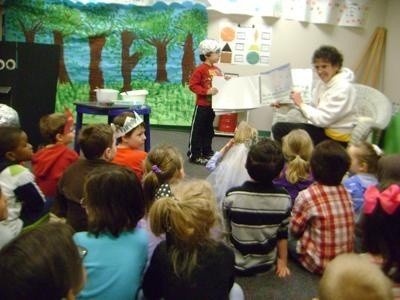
[312,65,329,72]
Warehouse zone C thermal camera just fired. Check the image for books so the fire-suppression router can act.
[211,62,312,117]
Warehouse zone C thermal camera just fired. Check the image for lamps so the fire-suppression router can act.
[347,84,394,145]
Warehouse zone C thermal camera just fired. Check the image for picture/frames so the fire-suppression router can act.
[73,101,152,156]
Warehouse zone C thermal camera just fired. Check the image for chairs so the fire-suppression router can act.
[203,150,216,159]
[190,155,209,165]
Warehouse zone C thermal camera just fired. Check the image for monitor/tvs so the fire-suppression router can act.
[211,62,312,117]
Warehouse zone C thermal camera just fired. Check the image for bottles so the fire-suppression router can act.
[199,39,221,55]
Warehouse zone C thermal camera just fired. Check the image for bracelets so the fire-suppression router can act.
[95,88,119,104]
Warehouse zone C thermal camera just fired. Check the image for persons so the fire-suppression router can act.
[186,39,233,166]
[2,105,400,300]
[268,42,357,149]
